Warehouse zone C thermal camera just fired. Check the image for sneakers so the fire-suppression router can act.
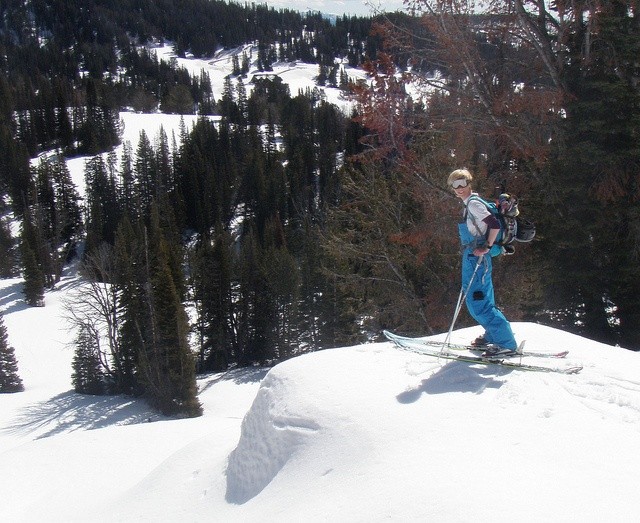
[481,344,516,356]
[471,335,490,346]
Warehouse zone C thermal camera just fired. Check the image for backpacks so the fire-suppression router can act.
[466,193,519,257]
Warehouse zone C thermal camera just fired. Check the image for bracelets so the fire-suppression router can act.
[481,242,492,250]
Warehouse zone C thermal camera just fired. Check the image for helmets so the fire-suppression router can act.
[516,217,536,242]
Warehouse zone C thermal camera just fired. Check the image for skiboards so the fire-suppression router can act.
[383,330,583,375]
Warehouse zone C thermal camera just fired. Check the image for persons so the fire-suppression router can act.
[447,169,517,358]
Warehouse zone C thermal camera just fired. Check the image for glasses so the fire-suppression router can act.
[452,179,471,189]
